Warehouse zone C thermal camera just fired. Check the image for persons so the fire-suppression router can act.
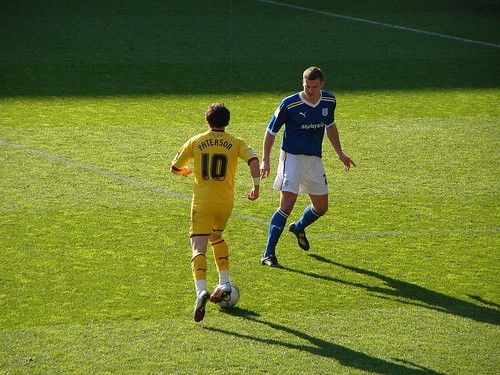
[258,67,358,267]
[171,102,261,324]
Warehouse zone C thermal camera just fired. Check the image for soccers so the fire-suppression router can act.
[217,281,239,309]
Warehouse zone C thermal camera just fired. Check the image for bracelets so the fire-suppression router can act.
[252,177,260,186]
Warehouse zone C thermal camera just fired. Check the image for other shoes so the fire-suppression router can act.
[288,222,310,251]
[194,288,211,322]
[208,283,232,304]
[260,253,285,269]
